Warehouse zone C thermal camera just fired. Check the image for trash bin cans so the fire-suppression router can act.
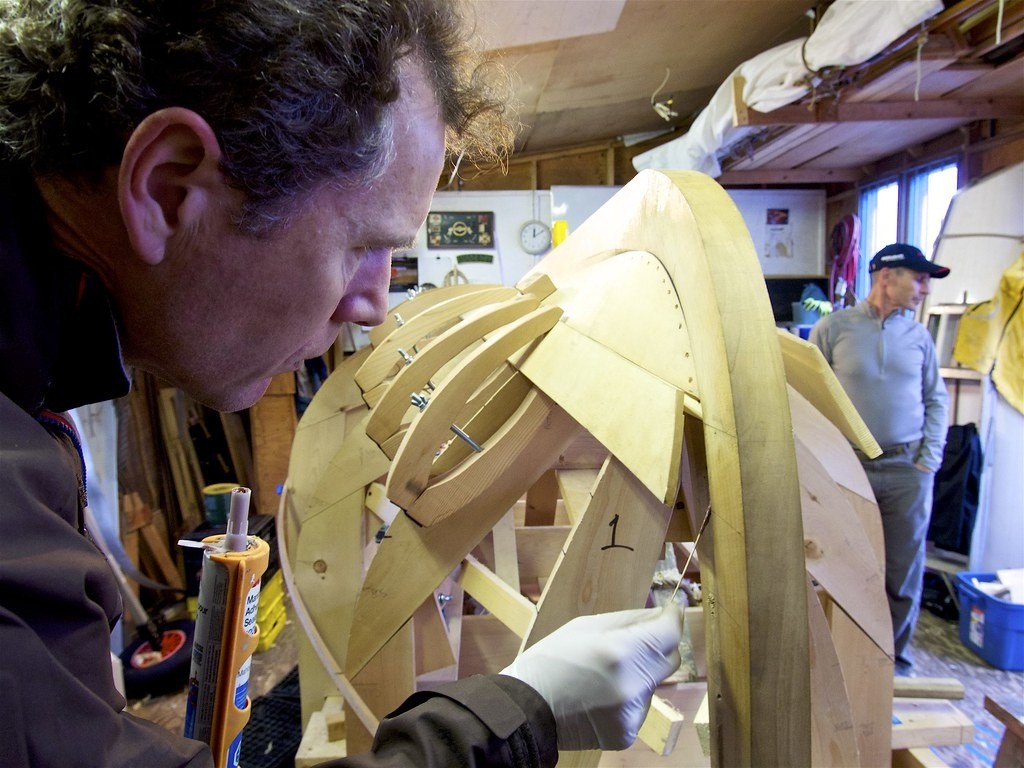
[956,572,1024,670]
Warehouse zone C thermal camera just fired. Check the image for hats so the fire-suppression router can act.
[870,242,951,280]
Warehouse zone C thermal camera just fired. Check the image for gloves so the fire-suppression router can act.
[501,603,690,752]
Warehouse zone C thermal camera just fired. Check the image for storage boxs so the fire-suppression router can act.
[956,571,1024,671]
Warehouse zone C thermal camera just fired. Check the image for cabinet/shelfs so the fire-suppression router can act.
[923,303,1004,584]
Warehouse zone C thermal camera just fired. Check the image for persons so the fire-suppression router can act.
[807,242,952,678]
[0,0,684,768]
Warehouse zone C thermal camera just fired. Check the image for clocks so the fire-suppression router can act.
[520,220,551,254]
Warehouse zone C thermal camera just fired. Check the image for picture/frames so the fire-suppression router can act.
[426,211,494,249]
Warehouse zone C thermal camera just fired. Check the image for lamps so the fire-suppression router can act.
[650,68,677,121]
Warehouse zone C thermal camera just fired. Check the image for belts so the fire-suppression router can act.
[854,440,924,463]
